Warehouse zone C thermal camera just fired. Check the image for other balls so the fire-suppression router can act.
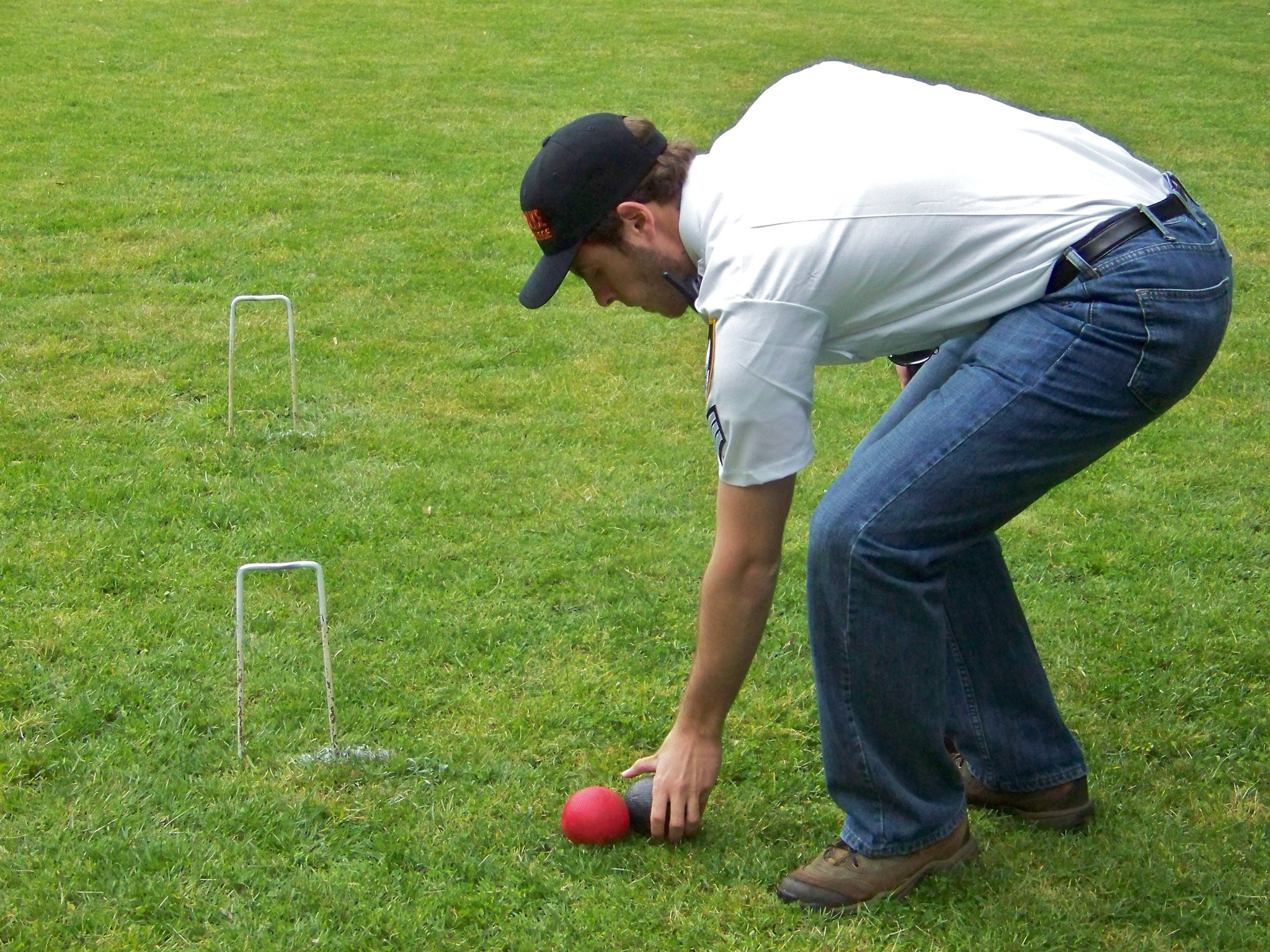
[624,776,671,838]
[563,785,630,845]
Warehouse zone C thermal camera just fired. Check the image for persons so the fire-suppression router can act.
[518,61,1233,909]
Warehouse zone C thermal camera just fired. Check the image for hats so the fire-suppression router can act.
[519,112,666,310]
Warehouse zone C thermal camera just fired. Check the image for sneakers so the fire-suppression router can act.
[778,810,974,914]
[951,751,1093,829]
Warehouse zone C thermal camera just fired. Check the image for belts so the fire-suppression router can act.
[1046,173,1188,294]
